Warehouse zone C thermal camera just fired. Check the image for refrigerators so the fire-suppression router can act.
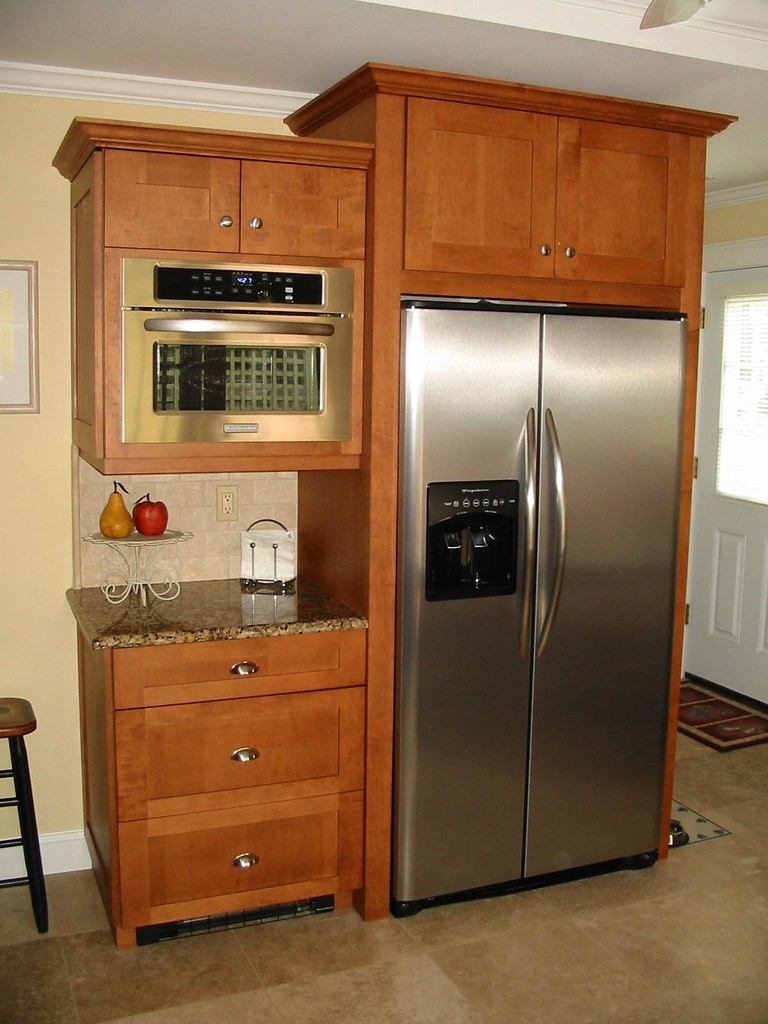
[391,294,689,918]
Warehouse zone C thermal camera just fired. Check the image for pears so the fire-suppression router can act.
[99,492,133,538]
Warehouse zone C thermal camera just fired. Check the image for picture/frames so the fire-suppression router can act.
[0,260,42,414]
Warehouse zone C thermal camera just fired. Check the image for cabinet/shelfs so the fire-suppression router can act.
[49,61,740,950]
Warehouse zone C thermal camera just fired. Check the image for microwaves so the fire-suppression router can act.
[118,255,355,446]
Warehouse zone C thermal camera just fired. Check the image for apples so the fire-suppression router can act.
[132,502,168,535]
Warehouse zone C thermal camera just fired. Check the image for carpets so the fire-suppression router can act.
[675,680,768,753]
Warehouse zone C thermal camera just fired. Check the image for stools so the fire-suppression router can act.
[0,697,51,935]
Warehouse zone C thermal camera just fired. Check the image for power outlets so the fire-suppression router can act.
[216,485,238,521]
